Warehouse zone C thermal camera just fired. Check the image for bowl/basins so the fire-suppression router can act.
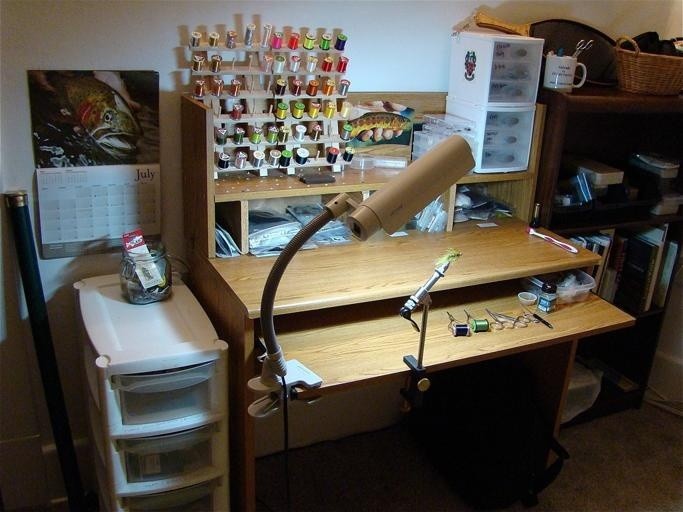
[515,291,537,306]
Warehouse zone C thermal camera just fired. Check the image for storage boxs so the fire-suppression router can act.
[443,94,535,174]
[447,32,546,107]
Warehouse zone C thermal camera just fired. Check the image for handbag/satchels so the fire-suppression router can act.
[398,356,570,511]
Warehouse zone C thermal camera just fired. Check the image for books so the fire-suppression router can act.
[570,224,677,312]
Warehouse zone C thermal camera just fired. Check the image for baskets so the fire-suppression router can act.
[613,36,682,96]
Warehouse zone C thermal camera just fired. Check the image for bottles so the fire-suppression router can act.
[537,282,559,314]
[118,238,172,306]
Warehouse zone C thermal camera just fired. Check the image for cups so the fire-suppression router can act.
[541,53,587,90]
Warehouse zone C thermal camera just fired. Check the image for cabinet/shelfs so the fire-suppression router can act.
[70,264,229,510]
[536,91,681,427]
[180,89,548,260]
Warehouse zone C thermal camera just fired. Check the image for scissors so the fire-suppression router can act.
[572,40,594,57]
[485,306,539,330]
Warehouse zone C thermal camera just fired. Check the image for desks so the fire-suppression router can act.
[201,220,636,511]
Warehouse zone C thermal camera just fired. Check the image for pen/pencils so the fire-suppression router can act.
[548,47,565,57]
[533,314,554,329]
